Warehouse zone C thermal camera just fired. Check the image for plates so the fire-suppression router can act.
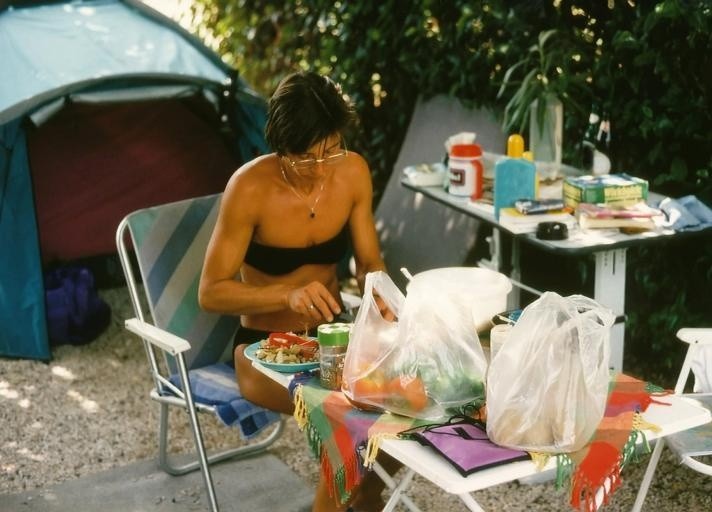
[243,337,320,372]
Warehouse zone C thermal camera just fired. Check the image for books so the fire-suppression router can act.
[562,171,648,213]
[578,200,661,230]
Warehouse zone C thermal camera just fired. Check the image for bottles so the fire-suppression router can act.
[589,111,617,176]
[448,143,484,198]
[317,320,351,391]
[494,134,538,224]
[578,103,600,172]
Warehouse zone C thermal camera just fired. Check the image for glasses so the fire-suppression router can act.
[283,132,349,169]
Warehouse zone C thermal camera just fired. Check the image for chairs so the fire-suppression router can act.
[631,327,712,512]
[115,190,286,511]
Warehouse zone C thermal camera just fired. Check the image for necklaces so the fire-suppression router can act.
[277,159,328,219]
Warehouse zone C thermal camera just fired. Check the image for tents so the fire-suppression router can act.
[0,1,269,367]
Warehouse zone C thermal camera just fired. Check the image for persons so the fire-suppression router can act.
[196,70,397,512]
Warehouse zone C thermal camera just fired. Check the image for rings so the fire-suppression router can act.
[306,304,314,311]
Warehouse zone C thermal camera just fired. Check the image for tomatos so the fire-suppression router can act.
[343,364,386,409]
[385,376,427,412]
